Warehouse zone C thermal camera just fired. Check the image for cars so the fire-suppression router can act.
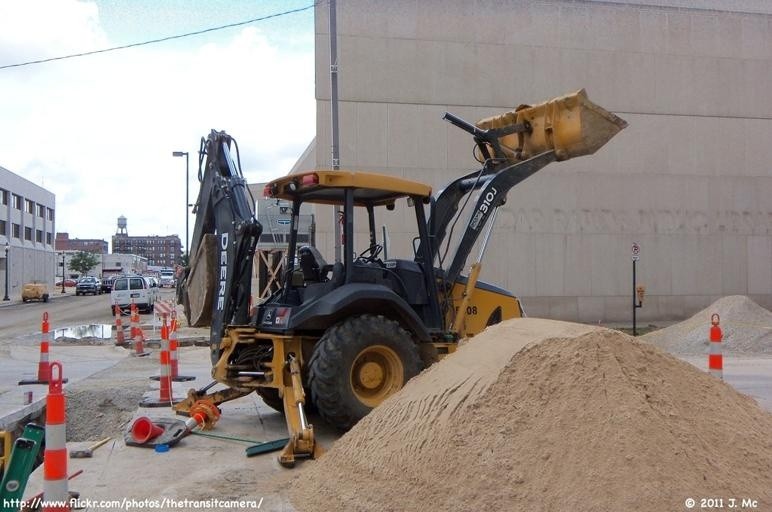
[57,275,113,296]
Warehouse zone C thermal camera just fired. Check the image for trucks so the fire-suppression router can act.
[158,266,176,288]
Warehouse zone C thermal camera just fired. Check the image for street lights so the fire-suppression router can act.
[2,239,12,301]
[61,250,67,294]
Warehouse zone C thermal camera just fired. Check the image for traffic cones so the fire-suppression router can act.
[708,313,725,386]
[115,299,176,445]
[39,360,72,512]
[37,309,54,382]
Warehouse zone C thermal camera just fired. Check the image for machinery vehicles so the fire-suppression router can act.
[167,85,633,470]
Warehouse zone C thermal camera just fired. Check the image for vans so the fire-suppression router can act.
[110,275,155,317]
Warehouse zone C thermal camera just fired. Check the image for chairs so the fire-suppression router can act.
[300,247,334,282]
